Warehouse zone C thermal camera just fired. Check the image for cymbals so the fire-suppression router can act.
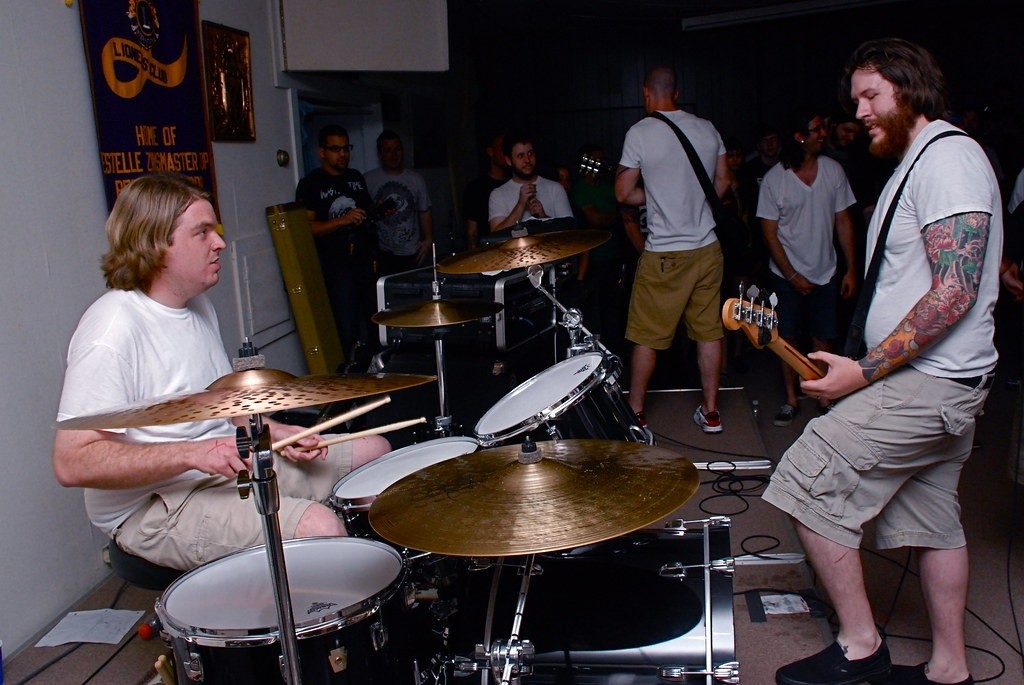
[48,366,439,431]
[371,298,504,328]
[435,227,613,274]
[367,438,700,558]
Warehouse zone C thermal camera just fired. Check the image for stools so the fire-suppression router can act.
[107,539,184,589]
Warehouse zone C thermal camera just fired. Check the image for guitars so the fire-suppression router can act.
[574,152,751,256]
[721,274,837,416]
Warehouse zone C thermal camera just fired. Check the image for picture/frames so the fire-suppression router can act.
[200,19,257,145]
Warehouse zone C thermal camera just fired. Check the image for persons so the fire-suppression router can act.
[760,39,1003,685]
[296,65,1024,433]
[52,173,393,571]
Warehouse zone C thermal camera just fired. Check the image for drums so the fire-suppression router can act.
[472,350,658,447]
[427,515,743,685]
[330,435,485,562]
[154,535,421,685]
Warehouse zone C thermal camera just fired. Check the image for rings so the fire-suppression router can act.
[817,396,820,400]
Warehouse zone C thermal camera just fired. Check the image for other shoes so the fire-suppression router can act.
[867,657,974,685]
[775,627,890,685]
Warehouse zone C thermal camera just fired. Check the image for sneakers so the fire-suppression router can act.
[637,413,647,428]
[773,406,799,427]
[693,404,722,433]
[813,403,832,417]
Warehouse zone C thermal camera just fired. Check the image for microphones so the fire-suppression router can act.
[528,196,539,219]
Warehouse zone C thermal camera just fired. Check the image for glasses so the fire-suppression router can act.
[804,123,827,134]
[322,144,354,153]
